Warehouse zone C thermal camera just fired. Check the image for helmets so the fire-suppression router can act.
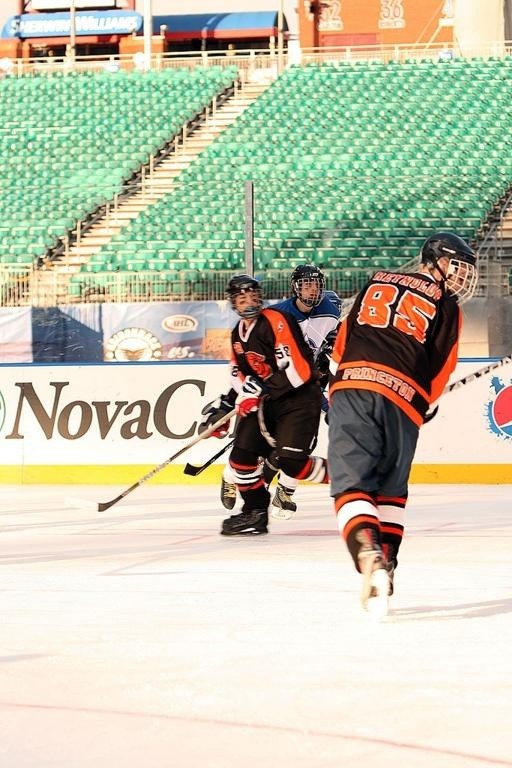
[422,233,479,306]
[290,265,326,307]
[225,275,264,318]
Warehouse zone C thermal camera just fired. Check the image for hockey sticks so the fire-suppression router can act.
[68,410,241,512]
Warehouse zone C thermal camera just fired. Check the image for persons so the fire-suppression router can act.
[318,233,478,620]
[221,264,343,511]
[218,273,330,533]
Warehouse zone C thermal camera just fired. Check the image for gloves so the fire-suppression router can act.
[207,396,234,438]
[234,375,263,417]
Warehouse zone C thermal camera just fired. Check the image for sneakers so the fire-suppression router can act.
[221,479,296,536]
[369,557,393,597]
